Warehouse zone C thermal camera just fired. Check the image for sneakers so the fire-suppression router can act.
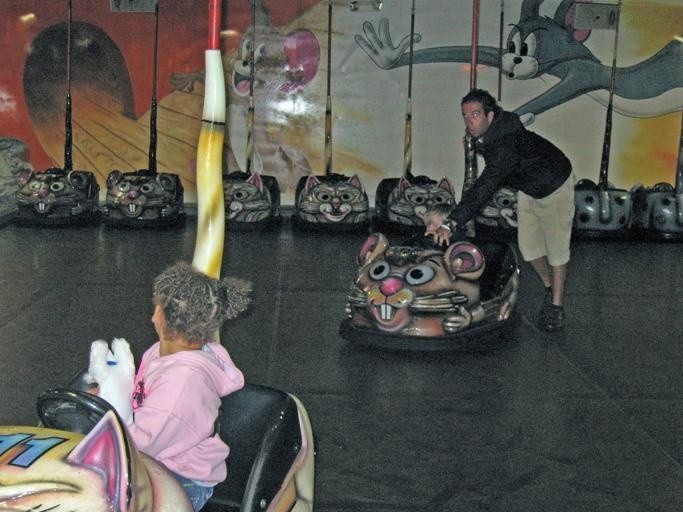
[541,285,555,307]
[544,305,565,334]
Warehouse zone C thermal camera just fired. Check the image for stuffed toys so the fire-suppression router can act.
[81,336,135,427]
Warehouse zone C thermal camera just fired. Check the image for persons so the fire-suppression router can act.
[426,205,452,240]
[88,260,254,511]
[433,89,577,331]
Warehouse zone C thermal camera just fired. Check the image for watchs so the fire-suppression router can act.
[441,221,455,230]
